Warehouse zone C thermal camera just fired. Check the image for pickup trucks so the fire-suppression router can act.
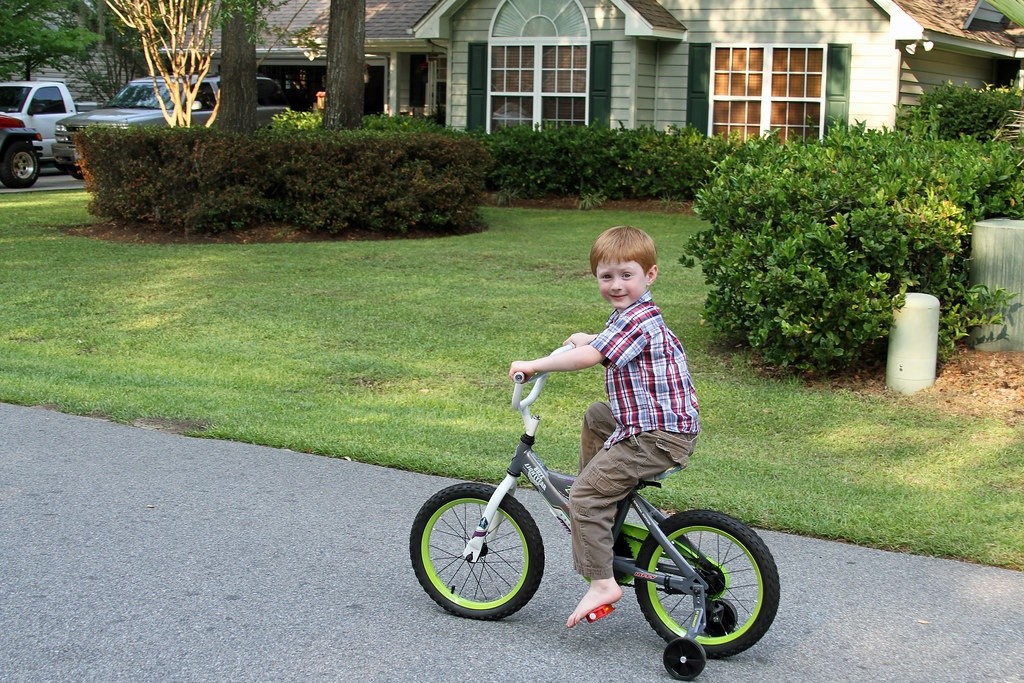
[0,80,106,171]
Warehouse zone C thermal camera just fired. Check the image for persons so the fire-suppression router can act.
[510,225,700,627]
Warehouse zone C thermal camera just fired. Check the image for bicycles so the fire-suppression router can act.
[407,342,782,682]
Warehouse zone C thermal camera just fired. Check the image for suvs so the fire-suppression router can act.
[51,73,291,180]
[0,112,45,189]
